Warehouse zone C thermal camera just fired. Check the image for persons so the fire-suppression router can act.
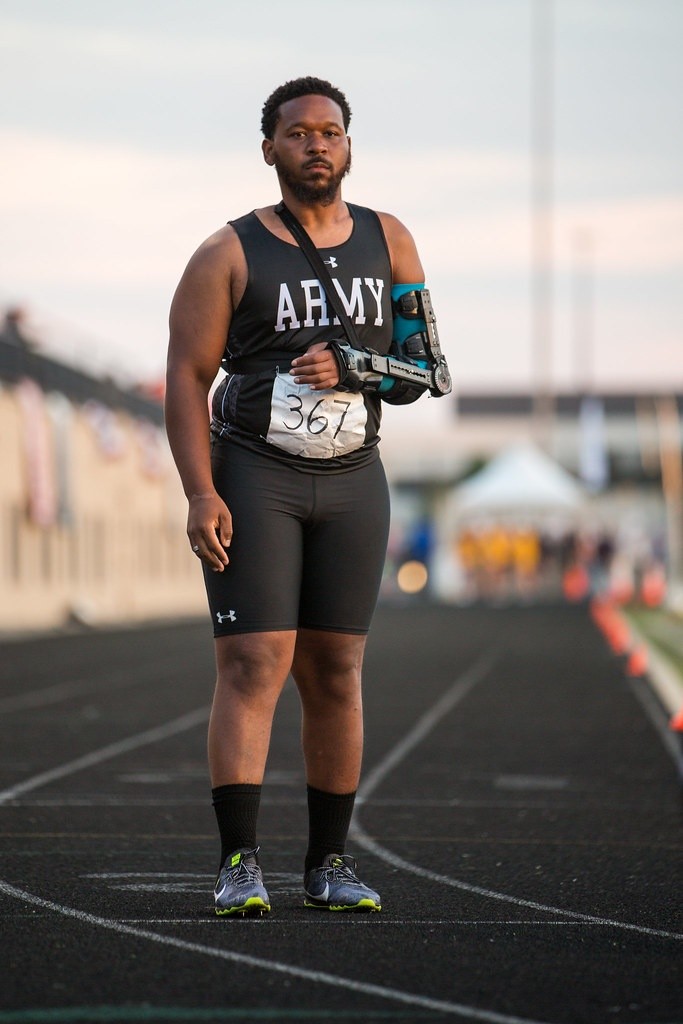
[165,76,454,914]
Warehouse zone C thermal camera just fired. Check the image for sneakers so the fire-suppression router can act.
[212,848,271,915]
[305,854,382,913]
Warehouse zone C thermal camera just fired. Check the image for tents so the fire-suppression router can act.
[433,442,589,605]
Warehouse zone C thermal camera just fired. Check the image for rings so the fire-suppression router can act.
[193,546,199,552]
